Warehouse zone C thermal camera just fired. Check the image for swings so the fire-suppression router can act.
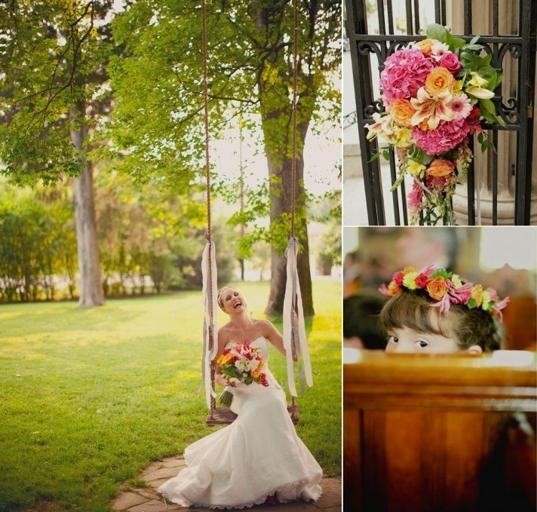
[202,0,298,426]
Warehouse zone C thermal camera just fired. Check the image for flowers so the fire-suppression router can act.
[216,340,264,407]
[378,262,512,323]
[364,23,508,225]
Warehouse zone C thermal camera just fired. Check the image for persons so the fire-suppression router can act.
[380,264,510,353]
[156,285,325,510]
